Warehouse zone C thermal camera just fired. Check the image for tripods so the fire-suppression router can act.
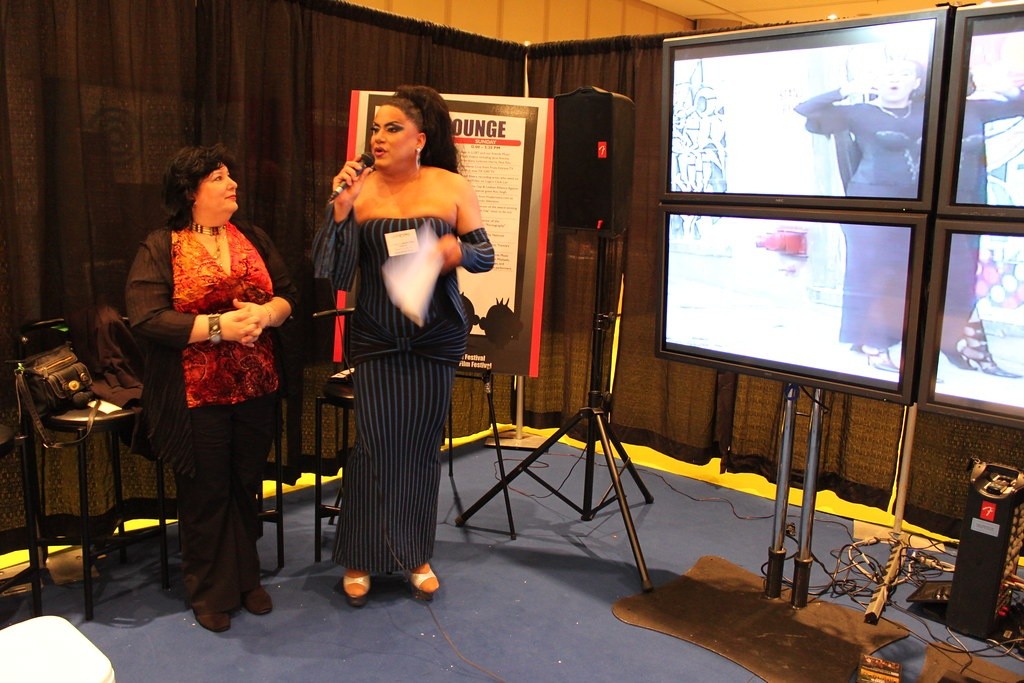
[456,234,653,592]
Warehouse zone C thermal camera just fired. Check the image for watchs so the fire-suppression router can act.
[209,312,221,343]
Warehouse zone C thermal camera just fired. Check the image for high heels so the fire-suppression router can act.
[343,568,371,606]
[406,564,440,601]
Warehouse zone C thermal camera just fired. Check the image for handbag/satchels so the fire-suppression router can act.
[15,346,101,450]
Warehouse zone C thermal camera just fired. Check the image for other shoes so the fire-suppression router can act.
[240,585,272,615]
[196,608,230,632]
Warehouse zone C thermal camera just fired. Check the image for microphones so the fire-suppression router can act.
[71,390,94,409]
[329,152,376,201]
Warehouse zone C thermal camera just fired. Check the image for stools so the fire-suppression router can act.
[314,308,362,562]
[11,316,169,620]
[0,425,42,617]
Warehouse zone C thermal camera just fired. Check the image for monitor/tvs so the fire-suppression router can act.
[663,2,1024,430]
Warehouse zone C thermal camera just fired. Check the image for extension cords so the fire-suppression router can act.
[911,552,938,567]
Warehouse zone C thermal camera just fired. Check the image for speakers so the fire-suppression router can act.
[555,86,636,236]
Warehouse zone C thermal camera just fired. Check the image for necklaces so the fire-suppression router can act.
[190,220,227,270]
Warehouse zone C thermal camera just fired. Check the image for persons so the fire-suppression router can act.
[314,83,496,606]
[128,143,297,632]
[791,55,1024,378]
